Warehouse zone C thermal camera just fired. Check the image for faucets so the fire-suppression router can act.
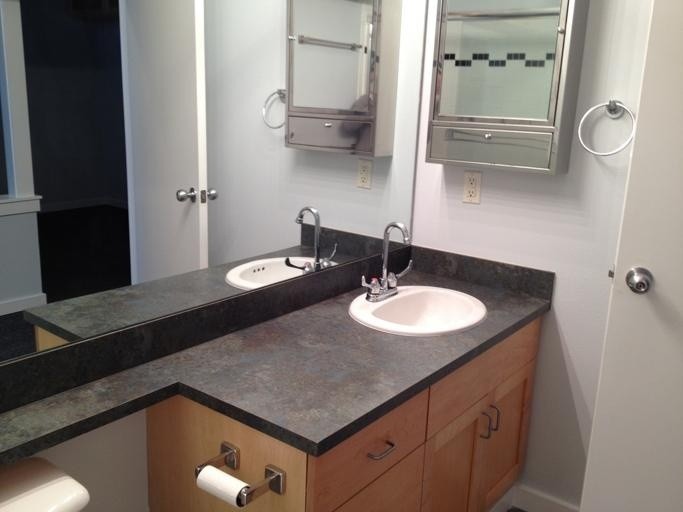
[294,205,321,267]
[378,221,411,290]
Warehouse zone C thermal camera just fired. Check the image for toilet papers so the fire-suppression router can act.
[195,462,249,509]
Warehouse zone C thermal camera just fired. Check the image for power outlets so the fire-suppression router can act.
[357,158,372,190]
[462,170,481,204]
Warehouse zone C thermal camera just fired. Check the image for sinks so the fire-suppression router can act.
[347,283,488,336]
[224,257,339,290]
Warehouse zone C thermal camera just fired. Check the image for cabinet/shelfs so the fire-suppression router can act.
[281,0,403,157]
[29,322,71,357]
[427,316,541,511]
[309,380,425,509]
[146,393,306,512]
[426,0,589,178]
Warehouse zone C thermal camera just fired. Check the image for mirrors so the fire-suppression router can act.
[1,0,429,413]
